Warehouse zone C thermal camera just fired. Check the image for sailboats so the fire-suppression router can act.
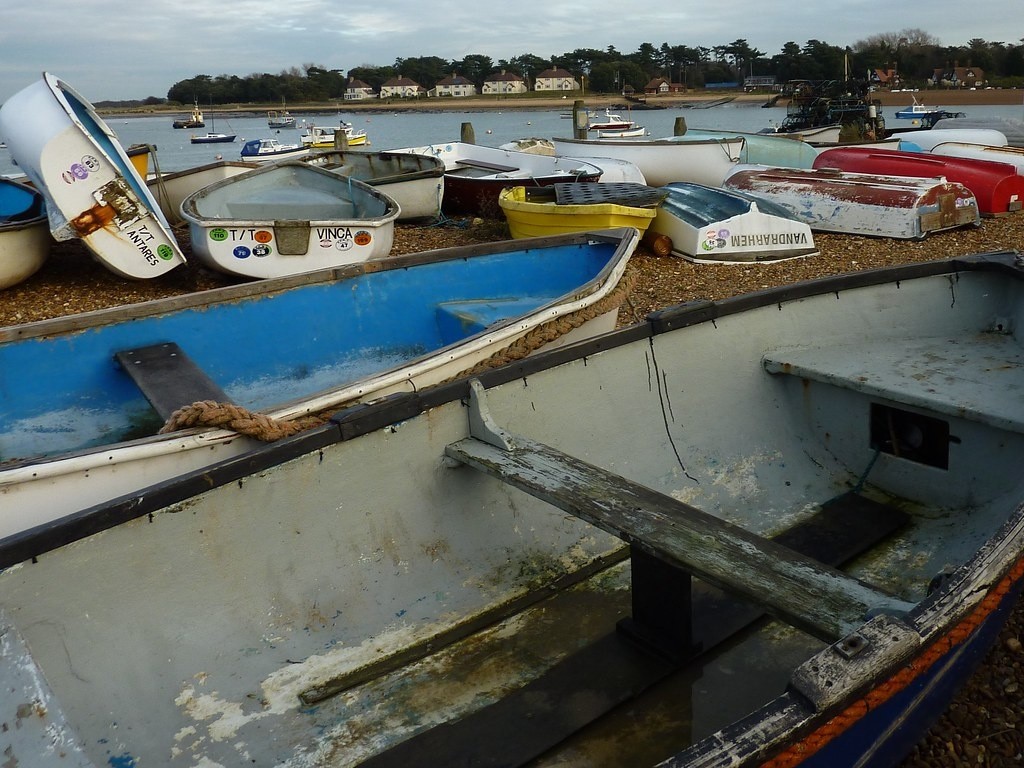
[190,91,237,143]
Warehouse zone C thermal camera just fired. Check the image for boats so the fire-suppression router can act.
[0,252,1024,768]
[588,115,634,131]
[553,137,744,188]
[650,181,818,264]
[497,183,658,247]
[0,143,157,187]
[172,95,206,129]
[604,114,620,118]
[299,119,367,148]
[0,72,191,285]
[146,162,266,225]
[756,50,902,154]
[267,97,296,128]
[241,138,311,163]
[0,227,641,535]
[0,176,52,291]
[180,160,402,279]
[559,109,595,119]
[380,141,604,221]
[812,146,1024,219]
[297,150,446,223]
[895,104,949,119]
[720,163,982,241]
[597,127,645,138]
[931,142,1024,177]
